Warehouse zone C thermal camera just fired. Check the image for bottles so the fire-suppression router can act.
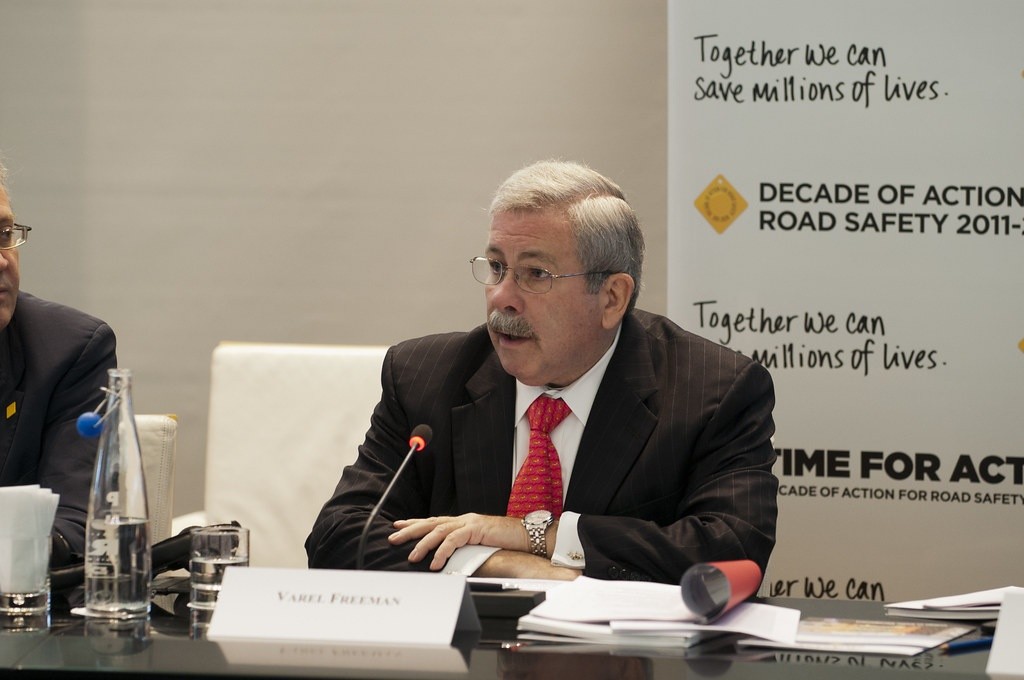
[84,366,155,627]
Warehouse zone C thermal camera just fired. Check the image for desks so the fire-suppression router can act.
[0,592,1024,680]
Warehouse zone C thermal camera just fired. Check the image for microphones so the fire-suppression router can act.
[356,424,433,569]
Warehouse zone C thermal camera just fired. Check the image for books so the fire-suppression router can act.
[882,586,1024,620]
[516,559,762,649]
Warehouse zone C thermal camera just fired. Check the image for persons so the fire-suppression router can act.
[304,161,781,600]
[0,179,119,565]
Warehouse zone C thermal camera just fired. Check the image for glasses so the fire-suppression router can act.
[0,221,33,251]
[469,256,617,294]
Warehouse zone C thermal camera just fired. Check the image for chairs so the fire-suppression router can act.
[132,413,178,547]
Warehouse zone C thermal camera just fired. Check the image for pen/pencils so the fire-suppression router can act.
[937,636,993,656]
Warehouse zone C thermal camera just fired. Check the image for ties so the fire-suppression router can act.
[506,394,573,522]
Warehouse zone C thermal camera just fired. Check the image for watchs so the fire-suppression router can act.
[520,509,555,559]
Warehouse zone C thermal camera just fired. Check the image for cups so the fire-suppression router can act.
[188,527,250,609]
[0,538,51,610]
